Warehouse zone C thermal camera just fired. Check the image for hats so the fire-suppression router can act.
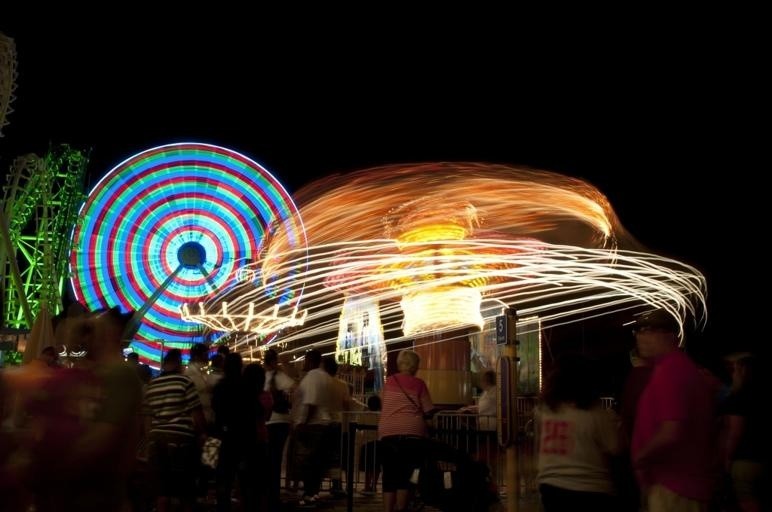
[632,308,679,332]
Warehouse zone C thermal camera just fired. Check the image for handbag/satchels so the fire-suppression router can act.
[271,388,292,411]
